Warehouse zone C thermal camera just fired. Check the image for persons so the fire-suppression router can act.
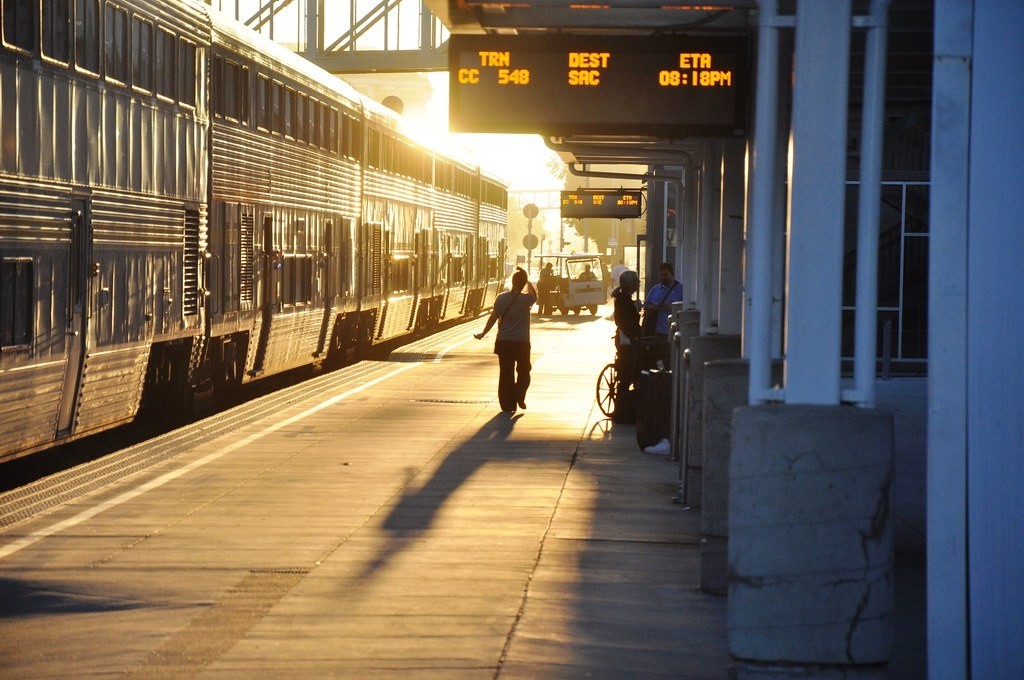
[578,264,598,280]
[611,259,629,289]
[473,266,538,412]
[537,262,554,315]
[610,270,641,393]
[642,263,684,369]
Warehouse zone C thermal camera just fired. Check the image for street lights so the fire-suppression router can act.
[540,233,545,271]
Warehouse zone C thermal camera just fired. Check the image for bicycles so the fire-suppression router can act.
[597,336,619,416]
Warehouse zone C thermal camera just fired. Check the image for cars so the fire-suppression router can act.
[505,262,513,278]
[589,262,612,278]
[527,269,540,283]
[550,266,567,279]
[571,263,584,276]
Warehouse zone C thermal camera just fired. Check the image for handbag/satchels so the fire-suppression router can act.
[494,334,500,355]
[641,312,657,337]
[619,329,630,346]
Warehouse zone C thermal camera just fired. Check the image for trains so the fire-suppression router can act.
[0,1,509,466]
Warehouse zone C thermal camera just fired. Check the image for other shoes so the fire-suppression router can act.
[517,396,526,409]
[645,439,671,454]
[614,416,636,424]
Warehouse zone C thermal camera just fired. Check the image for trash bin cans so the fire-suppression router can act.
[634,366,672,452]
